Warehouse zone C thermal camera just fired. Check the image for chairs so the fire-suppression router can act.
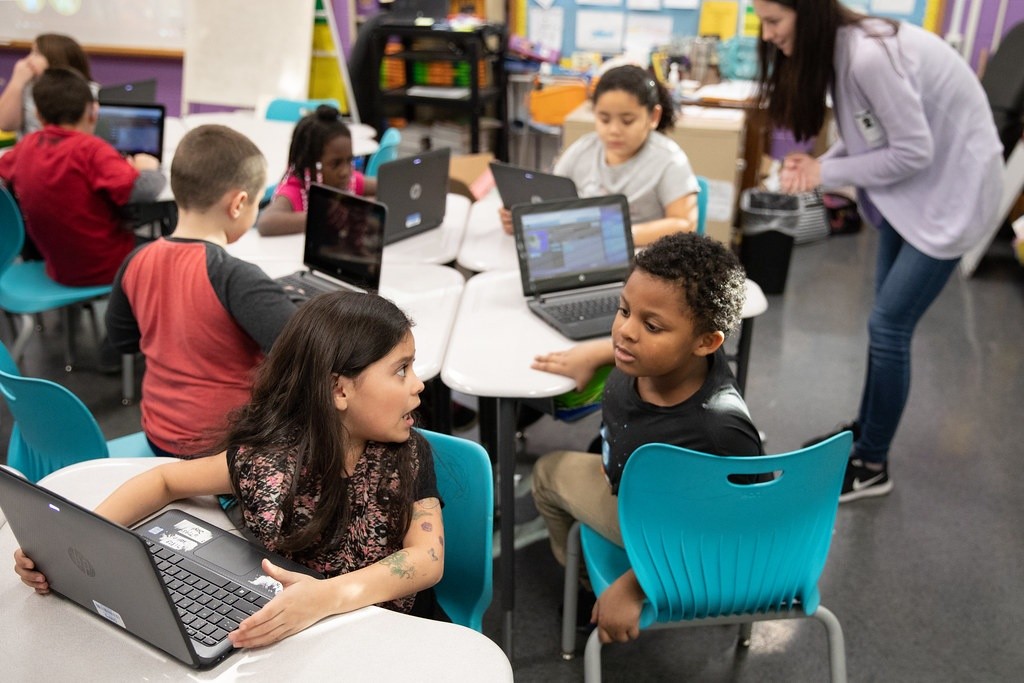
[263,97,340,125]
[1,184,123,373]
[1,341,162,486]
[364,126,401,178]
[413,424,497,631]
[559,429,854,683]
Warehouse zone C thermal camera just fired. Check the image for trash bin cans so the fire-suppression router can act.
[740,189,804,298]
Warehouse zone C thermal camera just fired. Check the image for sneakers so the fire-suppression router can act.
[802,425,860,459]
[838,460,892,503]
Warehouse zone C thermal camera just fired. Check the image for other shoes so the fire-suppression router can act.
[447,401,478,432]
[557,589,596,632]
[93,337,122,372]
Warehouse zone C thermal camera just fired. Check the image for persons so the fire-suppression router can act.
[14,291,455,648]
[530,232,777,645]
[0,66,166,376]
[753,0,1006,503]
[104,125,300,461]
[0,34,102,137]
[499,64,702,247]
[258,105,379,238]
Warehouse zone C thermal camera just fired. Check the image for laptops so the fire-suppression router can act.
[93,80,166,167]
[378,146,452,245]
[273,183,388,311]
[0,465,327,673]
[489,159,638,340]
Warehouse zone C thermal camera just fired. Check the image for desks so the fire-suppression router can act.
[139,111,377,233]
[1,228,770,683]
